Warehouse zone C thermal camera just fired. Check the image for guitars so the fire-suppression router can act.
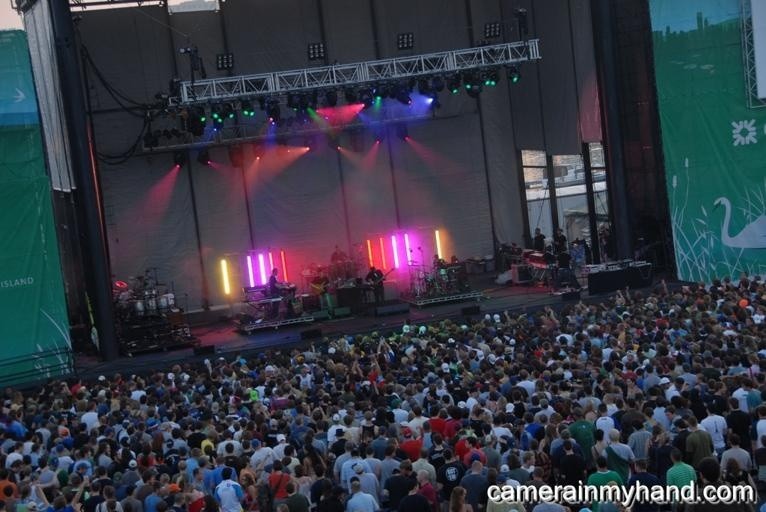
[309,281,330,295]
[369,268,396,290]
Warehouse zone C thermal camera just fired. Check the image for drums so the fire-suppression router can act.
[130,300,145,315]
[158,295,171,309]
[146,298,156,312]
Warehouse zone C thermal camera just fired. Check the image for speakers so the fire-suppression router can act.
[194,344,215,357]
[461,306,480,316]
[561,291,580,301]
[301,328,321,340]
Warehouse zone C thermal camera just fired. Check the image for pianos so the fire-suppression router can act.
[242,286,266,294]
[242,297,272,306]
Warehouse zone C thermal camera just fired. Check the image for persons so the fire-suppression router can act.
[1,228,766,512]
